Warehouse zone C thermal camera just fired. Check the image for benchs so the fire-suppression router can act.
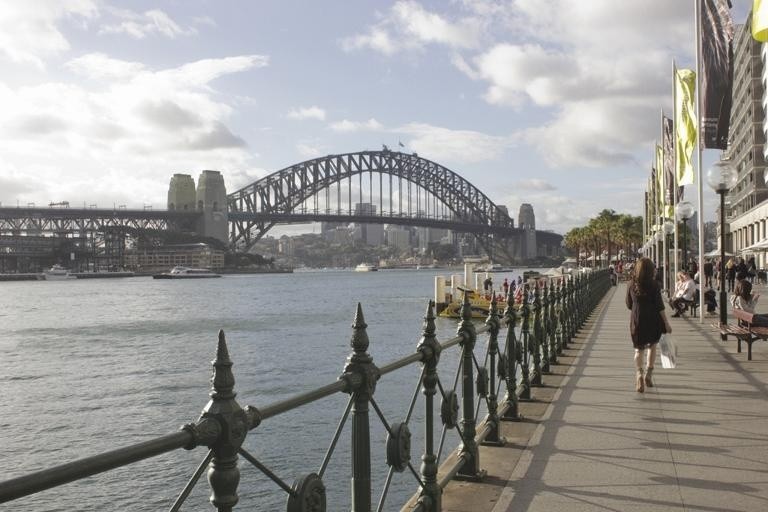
[710,309,768,361]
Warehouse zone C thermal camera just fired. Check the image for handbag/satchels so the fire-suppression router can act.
[658,332,679,369]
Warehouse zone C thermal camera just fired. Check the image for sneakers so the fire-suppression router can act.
[705,284,735,293]
[671,307,719,317]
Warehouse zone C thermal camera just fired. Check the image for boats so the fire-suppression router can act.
[151,264,223,279]
[472,264,514,272]
[42,262,79,282]
[356,262,378,272]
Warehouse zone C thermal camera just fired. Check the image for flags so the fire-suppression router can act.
[399,142,404,147]
[645,117,684,239]
[698,0,735,150]
[675,68,698,187]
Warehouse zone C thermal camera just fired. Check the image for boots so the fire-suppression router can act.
[636,368,645,394]
[644,366,654,388]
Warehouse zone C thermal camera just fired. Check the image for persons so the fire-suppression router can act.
[626,258,672,392]
[608,257,663,290]
[730,281,768,326]
[669,257,756,317]
[484,277,492,290]
[503,276,522,298]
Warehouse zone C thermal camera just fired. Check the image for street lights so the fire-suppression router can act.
[601,250,608,269]
[703,159,739,341]
[619,248,624,261]
[638,201,696,299]
